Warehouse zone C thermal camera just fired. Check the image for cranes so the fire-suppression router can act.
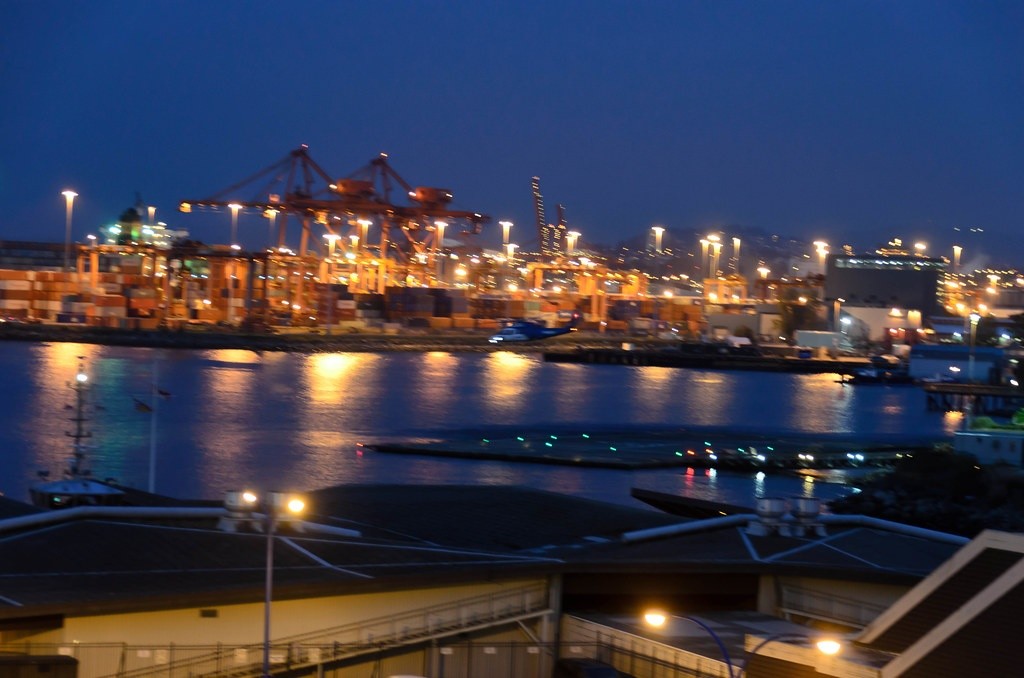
[172,143,568,260]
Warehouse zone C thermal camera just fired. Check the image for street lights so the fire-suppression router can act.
[652,227,666,253]
[60,189,79,271]
[239,492,308,678]
[757,267,772,280]
[498,221,514,248]
[711,242,724,274]
[434,219,448,254]
[643,608,844,678]
[226,200,243,257]
[954,246,964,273]
[732,237,741,273]
[700,238,713,273]
[357,219,373,248]
[502,240,520,260]
[322,232,342,259]
[814,240,833,263]
[568,231,584,247]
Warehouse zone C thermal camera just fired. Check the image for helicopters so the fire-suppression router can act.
[487,298,588,347]
